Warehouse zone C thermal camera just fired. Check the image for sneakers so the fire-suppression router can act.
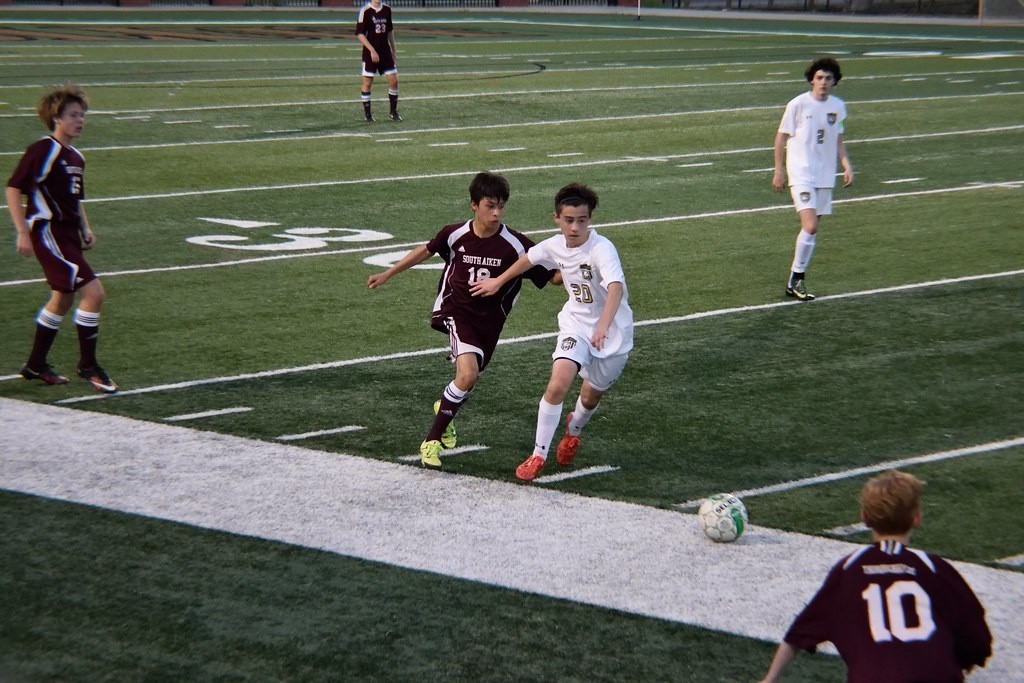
[76,361,118,394]
[434,400,457,449]
[556,411,580,465]
[388,112,403,122]
[20,365,69,385]
[365,116,375,122]
[420,438,445,468]
[786,280,815,302]
[515,454,546,481]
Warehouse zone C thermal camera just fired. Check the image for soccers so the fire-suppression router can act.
[699,493,751,542]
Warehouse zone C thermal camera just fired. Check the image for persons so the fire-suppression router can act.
[5,79,122,394]
[771,56,853,303]
[358,0,403,121]
[467,182,634,481]
[759,470,996,683]
[367,167,564,472]
